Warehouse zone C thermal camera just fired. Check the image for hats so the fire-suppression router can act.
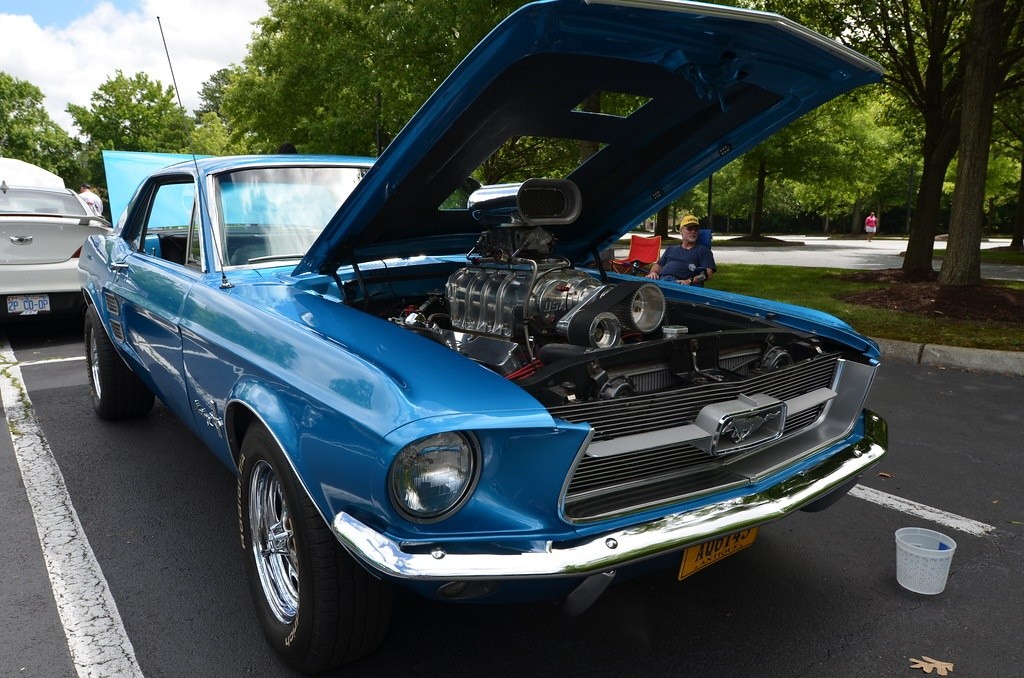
[681,215,699,227]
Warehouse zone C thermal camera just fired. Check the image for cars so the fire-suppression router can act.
[0,157,110,336]
[73,2,898,678]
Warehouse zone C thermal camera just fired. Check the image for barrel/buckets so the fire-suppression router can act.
[894,525,958,595]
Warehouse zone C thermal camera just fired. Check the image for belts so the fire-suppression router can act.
[660,274,680,280]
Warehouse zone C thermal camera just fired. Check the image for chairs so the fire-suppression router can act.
[611,234,662,276]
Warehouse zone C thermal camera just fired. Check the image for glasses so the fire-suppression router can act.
[683,227,700,231]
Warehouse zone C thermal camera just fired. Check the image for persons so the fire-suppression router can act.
[644,212,718,288]
[864,212,879,244]
[77,183,104,219]
[88,186,103,211]
